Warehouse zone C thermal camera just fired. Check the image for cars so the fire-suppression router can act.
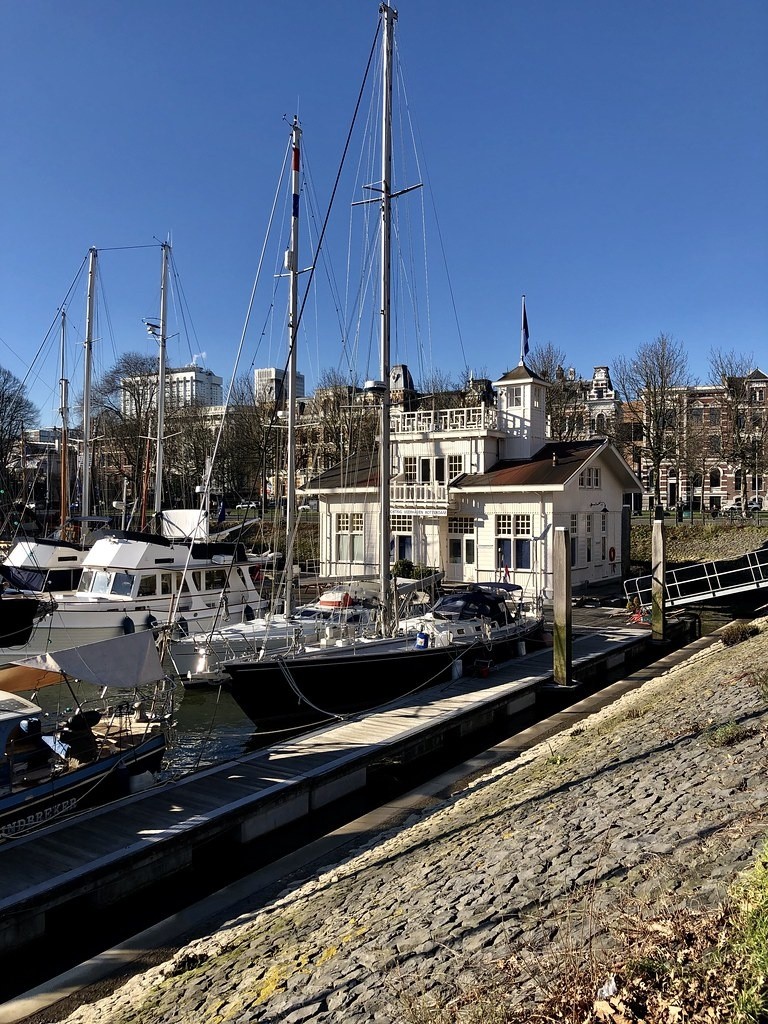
[297,504,310,512]
[67,501,80,510]
[25,502,45,510]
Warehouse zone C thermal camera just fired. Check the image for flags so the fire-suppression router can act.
[217,500,225,526]
[523,297,530,357]
[505,565,510,580]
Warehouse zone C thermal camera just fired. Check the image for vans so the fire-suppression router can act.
[667,501,706,514]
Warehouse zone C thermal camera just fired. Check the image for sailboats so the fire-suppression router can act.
[1,0,549,842]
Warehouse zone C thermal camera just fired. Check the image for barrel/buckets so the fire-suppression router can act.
[416,632,428,648]
[212,555,235,564]
[480,666,489,678]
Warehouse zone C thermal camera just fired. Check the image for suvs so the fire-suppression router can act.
[722,500,761,512]
[235,501,261,511]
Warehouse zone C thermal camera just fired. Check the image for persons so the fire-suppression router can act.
[631,597,641,614]
[675,497,684,522]
[710,504,718,520]
